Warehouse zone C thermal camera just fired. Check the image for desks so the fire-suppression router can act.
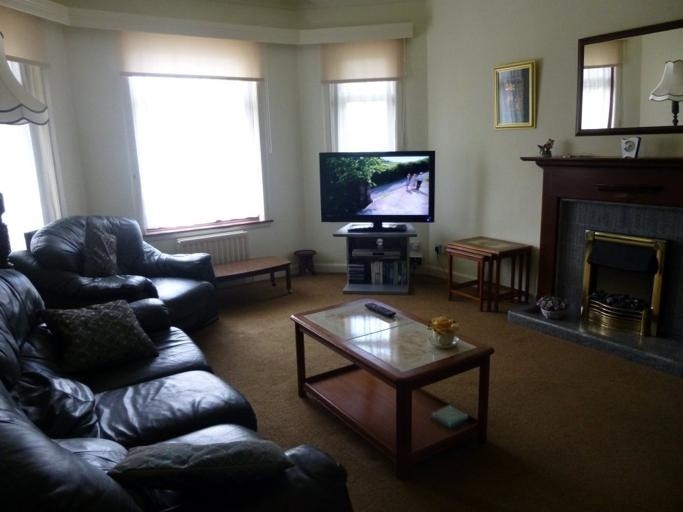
[446,236,531,312]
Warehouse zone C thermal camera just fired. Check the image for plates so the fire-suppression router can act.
[426,337,461,348]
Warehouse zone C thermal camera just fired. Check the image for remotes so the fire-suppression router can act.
[365,303,396,316]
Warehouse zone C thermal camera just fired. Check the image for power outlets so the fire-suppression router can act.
[435,242,445,256]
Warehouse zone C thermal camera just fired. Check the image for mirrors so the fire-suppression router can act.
[575,19,683,136]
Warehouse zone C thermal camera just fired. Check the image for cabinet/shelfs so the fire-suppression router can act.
[332,222,417,295]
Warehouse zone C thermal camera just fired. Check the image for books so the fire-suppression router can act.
[349,258,408,287]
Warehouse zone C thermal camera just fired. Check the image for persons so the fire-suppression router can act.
[415,171,423,191]
[404,173,410,190]
[410,174,415,190]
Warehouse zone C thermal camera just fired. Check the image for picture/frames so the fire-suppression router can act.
[492,59,537,129]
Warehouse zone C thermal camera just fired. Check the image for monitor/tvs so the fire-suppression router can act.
[319,151,435,232]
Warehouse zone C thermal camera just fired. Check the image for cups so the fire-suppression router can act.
[432,329,456,345]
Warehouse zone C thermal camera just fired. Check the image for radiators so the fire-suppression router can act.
[176,230,254,289]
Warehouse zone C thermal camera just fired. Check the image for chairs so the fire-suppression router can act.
[9,214,219,340]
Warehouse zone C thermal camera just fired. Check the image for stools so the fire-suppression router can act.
[295,250,317,278]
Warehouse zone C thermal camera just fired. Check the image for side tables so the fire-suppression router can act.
[213,256,293,303]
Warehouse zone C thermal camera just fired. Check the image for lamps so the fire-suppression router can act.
[0,32,50,268]
[648,59,683,129]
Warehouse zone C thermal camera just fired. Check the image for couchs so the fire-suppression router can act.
[1,264,354,510]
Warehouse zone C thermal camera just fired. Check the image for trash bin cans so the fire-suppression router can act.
[295,250,315,274]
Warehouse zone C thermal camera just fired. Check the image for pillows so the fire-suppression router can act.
[81,216,151,280]
[37,299,159,384]
[106,440,295,486]
